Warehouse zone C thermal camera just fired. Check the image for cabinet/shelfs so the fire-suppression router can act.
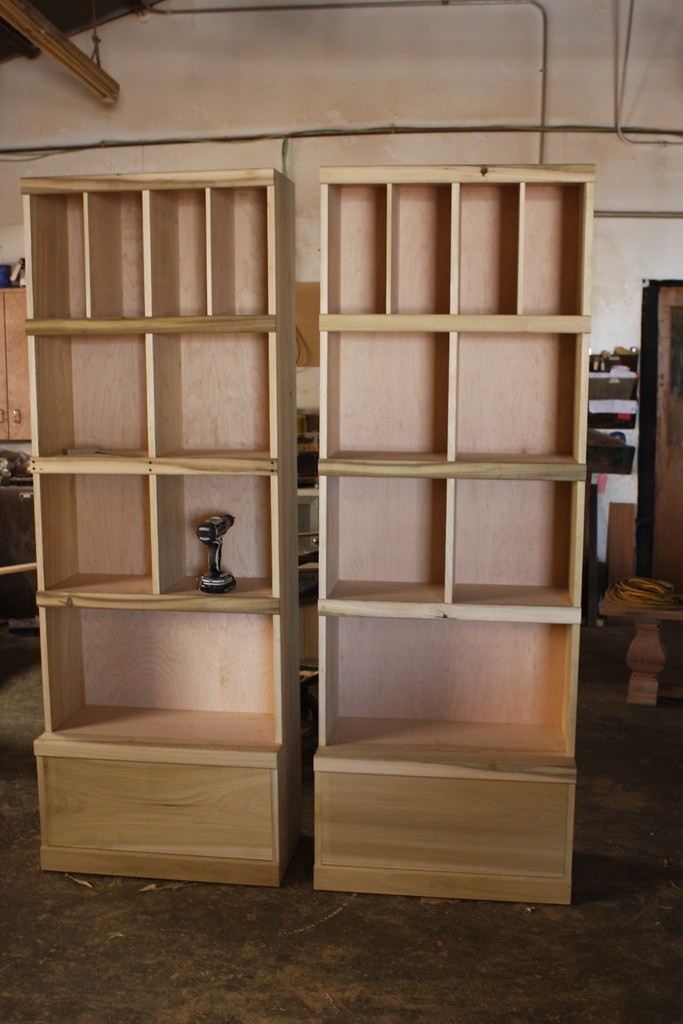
[312,164,596,905]
[0,287,31,441]
[20,169,302,886]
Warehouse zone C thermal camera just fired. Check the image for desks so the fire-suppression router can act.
[0,485,40,635]
[599,594,683,706]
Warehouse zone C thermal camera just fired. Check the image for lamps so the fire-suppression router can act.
[0,0,119,103]
